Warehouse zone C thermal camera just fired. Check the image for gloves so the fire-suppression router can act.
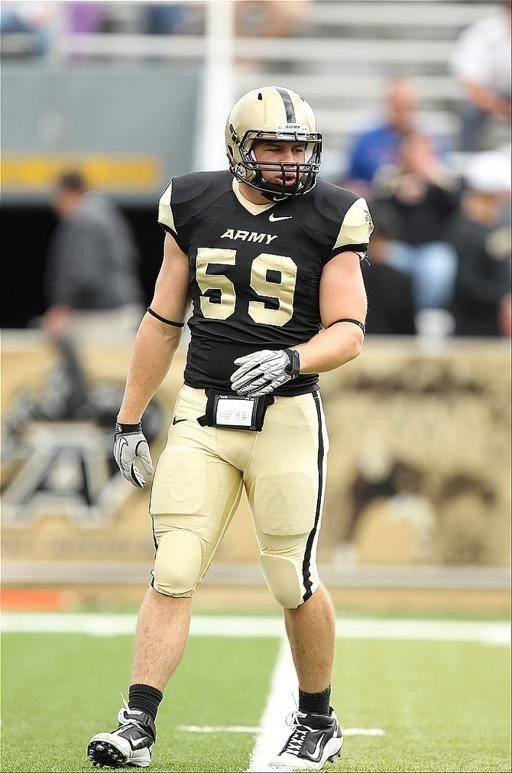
[229,349,301,398]
[114,423,153,488]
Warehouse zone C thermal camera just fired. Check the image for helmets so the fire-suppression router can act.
[224,88,323,199]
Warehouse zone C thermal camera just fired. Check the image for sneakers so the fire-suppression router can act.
[270,707,342,768]
[87,708,157,767]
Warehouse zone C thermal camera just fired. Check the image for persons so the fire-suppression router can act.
[87,89,371,773]
